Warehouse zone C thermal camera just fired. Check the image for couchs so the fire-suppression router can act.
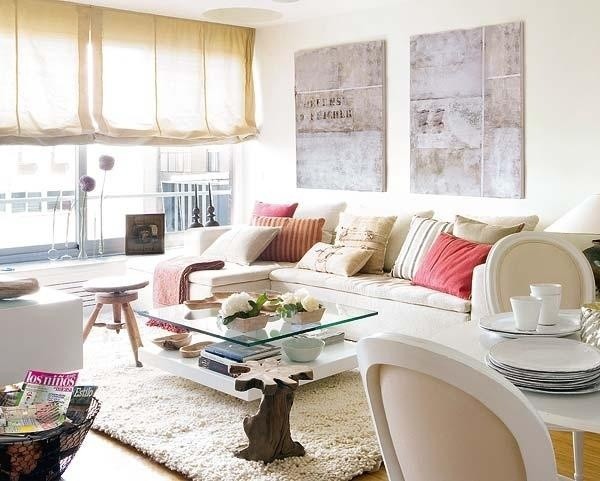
[128,195,600,343]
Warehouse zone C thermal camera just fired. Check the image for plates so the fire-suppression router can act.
[479,313,600,396]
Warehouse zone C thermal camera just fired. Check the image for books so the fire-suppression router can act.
[288,328,345,346]
[0,370,98,437]
[199,335,282,378]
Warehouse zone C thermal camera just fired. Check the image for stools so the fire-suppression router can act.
[228,356,313,464]
[83,277,149,367]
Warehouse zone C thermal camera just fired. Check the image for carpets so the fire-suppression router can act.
[72,354,382,481]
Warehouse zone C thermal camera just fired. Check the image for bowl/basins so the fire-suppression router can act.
[282,337,325,363]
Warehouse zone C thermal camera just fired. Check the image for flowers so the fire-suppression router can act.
[99,155,114,253]
[79,176,95,256]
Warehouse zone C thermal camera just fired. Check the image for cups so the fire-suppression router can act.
[510,295,541,331]
[581,302,600,350]
[531,283,562,325]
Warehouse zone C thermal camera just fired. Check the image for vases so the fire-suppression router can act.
[98,194,105,257]
[79,193,87,260]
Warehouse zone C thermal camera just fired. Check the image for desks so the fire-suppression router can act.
[0,285,83,386]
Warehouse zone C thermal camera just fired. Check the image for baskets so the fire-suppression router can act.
[0,391,101,481]
